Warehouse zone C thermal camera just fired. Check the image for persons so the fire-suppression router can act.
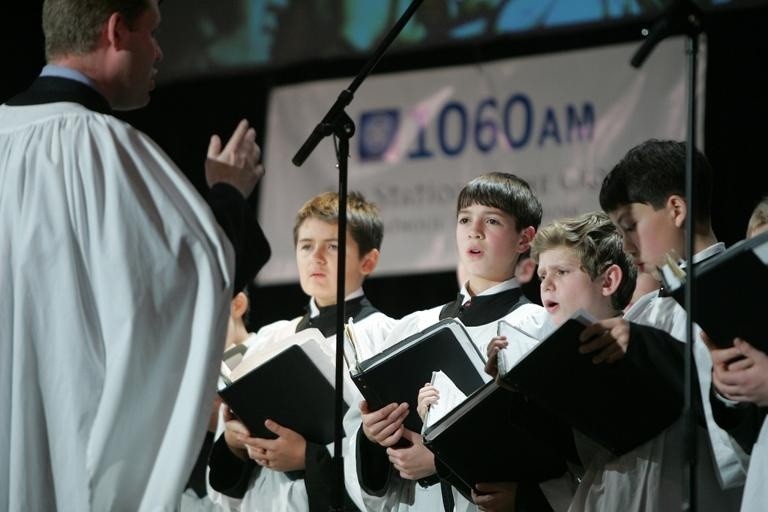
[183,138,767,511]
[0,1,271,512]
